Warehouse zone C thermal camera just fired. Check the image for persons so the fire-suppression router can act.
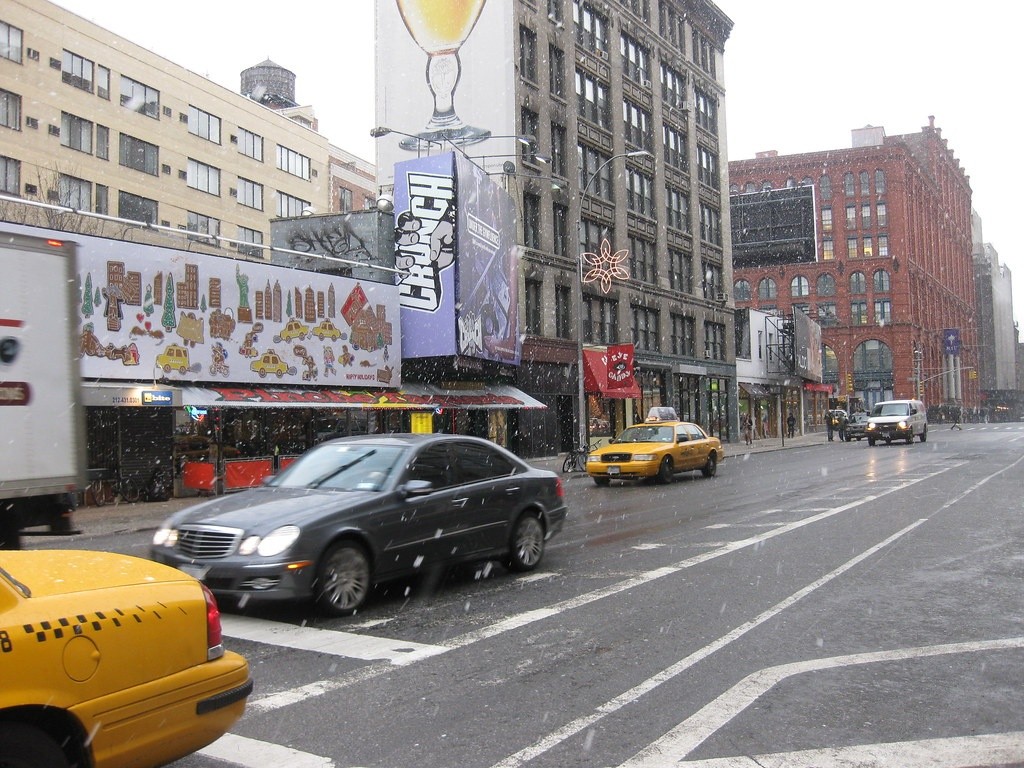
[824,410,847,441]
[786,412,797,438]
[743,414,753,446]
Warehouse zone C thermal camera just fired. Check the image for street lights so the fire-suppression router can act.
[575,149,651,447]
[370,126,568,191]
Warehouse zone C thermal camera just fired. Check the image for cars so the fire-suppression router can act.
[585,407,725,486]
[150,432,569,617]
[0,548,256,768]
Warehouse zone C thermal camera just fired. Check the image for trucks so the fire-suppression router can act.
[0,230,90,552]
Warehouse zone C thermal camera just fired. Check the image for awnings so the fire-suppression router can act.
[582,348,641,398]
[175,383,548,409]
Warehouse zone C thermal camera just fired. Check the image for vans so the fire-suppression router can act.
[829,409,848,417]
[867,399,928,446]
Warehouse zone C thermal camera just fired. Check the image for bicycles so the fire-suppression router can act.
[76,457,189,507]
[560,437,602,472]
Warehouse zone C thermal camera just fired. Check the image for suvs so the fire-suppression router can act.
[845,411,869,441]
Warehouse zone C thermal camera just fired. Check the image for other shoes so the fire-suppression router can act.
[750,441,752,444]
[792,436,793,438]
[746,442,749,445]
[828,439,834,441]
[788,436,790,438]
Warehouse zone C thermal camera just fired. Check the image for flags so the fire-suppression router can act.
[943,328,960,356]
[606,344,634,391]
